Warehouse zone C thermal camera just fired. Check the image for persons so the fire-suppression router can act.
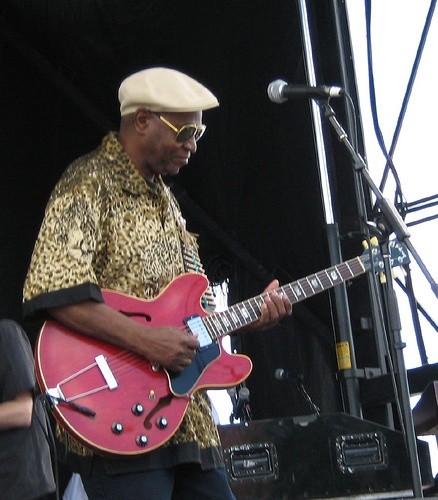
[21,66,293,500]
[0,318,56,499]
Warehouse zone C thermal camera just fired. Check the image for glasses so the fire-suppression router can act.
[155,111,206,143]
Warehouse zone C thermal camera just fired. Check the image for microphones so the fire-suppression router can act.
[267,79,345,104]
[274,369,304,382]
[234,388,250,420]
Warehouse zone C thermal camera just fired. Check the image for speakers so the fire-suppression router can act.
[217,412,433,500]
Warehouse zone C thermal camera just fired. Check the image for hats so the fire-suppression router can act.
[117,67,220,117]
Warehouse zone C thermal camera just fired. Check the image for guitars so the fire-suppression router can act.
[34,236,412,457]
